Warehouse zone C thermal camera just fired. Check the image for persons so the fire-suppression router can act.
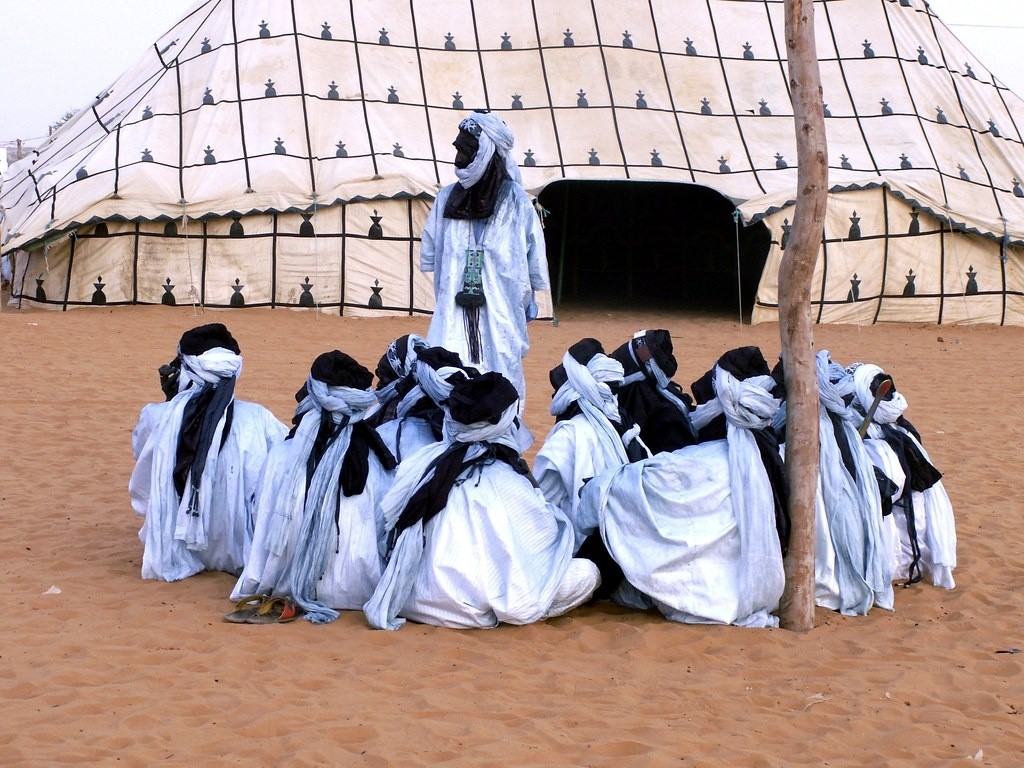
[420,113,553,418]
[129,324,958,627]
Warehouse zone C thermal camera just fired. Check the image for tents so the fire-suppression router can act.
[0,0,1023,325]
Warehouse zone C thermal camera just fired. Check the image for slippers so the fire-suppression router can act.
[225,595,270,623]
[247,598,296,624]
